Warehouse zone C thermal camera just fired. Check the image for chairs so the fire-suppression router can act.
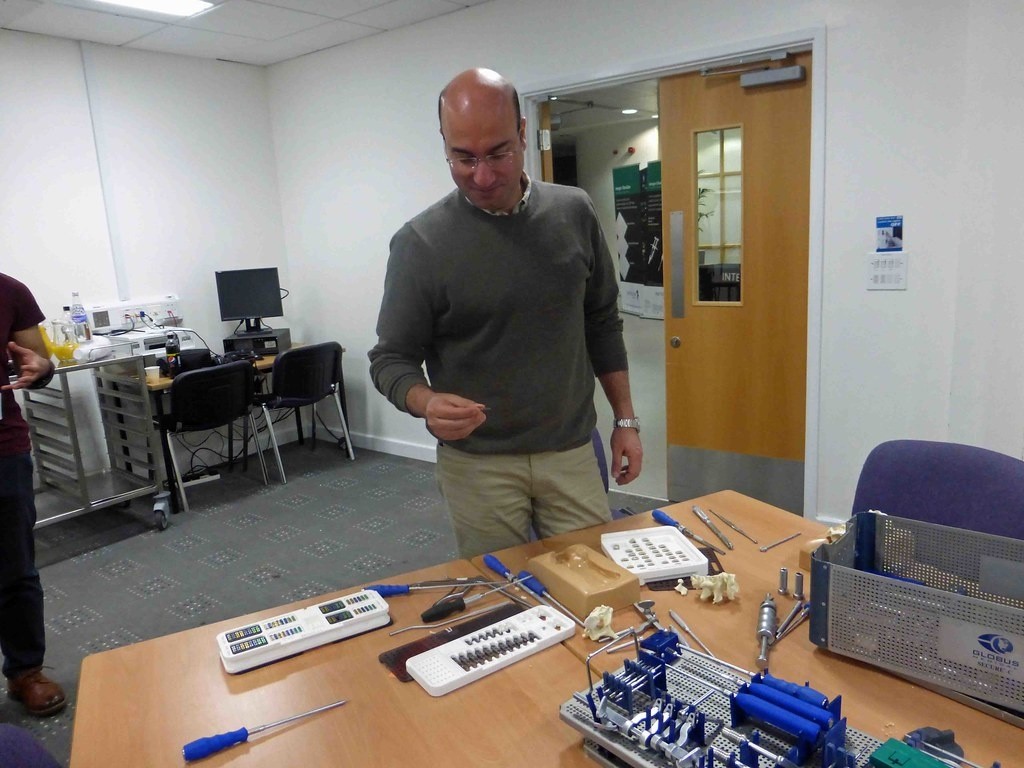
[254,342,355,484]
[152,360,268,514]
[851,439,1024,543]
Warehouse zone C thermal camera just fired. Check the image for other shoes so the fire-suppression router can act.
[7,666,67,716]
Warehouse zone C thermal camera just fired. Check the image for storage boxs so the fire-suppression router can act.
[809,513,1024,728]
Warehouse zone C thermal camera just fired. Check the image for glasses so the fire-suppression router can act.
[443,128,521,169]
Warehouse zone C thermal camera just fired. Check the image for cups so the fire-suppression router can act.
[144,366,160,384]
[52,319,78,367]
[38,322,53,359]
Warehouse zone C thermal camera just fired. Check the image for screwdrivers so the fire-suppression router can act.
[362,580,509,598]
[483,553,585,628]
[651,509,727,556]
[771,599,811,645]
[661,643,835,744]
[181,698,352,764]
[420,574,533,625]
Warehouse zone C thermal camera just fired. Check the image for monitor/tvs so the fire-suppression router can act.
[215,267,283,337]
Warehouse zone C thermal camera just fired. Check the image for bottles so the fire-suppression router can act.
[61,305,72,322]
[71,290,92,343]
[166,334,182,378]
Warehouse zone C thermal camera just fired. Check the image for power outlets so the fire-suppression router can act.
[165,303,178,319]
[119,307,135,326]
[135,308,148,326]
[148,304,165,321]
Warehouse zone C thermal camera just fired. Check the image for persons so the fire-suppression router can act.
[0,271,68,717]
[367,68,643,562]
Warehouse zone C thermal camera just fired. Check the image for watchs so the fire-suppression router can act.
[614,416,640,433]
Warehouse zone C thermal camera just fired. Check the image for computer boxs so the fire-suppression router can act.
[223,328,291,355]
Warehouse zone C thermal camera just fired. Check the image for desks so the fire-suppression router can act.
[68,489,1024,768]
[133,342,351,514]
[9,352,171,530]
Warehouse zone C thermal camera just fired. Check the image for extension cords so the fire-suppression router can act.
[174,473,220,486]
